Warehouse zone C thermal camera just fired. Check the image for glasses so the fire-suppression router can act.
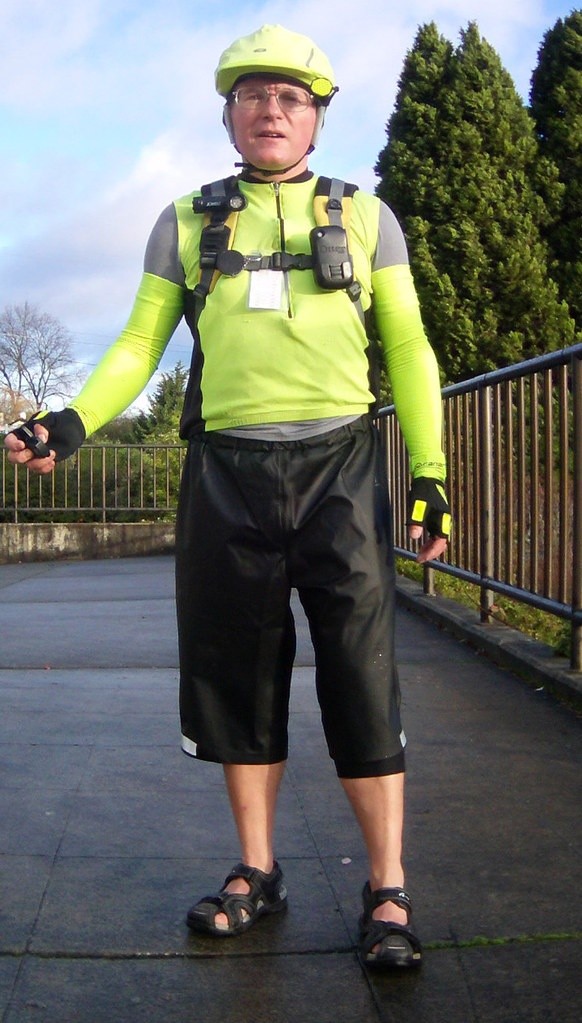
[229,85,315,112]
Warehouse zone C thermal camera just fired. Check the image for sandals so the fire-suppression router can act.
[185,860,288,936]
[358,879,423,969]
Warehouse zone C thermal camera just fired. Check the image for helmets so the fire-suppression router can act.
[214,23,339,107]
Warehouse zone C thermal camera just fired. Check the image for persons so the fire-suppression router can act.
[4,23,456,969]
